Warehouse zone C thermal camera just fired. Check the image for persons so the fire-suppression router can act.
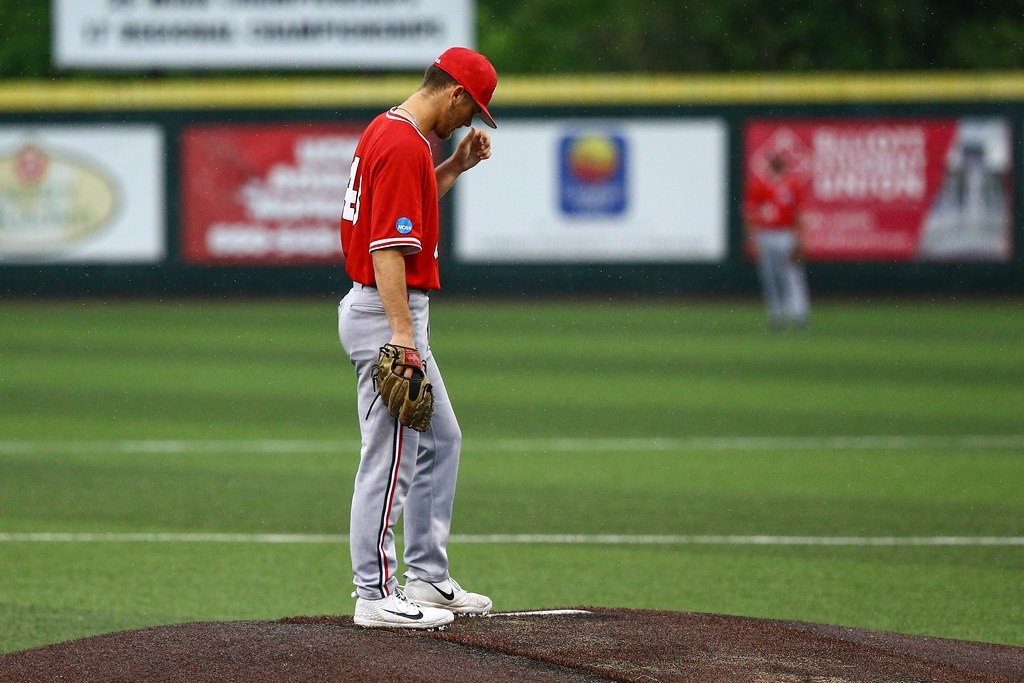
[337,45,501,629]
[746,154,810,330]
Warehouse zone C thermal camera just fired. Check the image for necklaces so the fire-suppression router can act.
[397,107,420,128]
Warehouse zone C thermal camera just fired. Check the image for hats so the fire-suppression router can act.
[433,46,497,129]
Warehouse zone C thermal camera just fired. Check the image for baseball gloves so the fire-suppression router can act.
[364,342,436,433]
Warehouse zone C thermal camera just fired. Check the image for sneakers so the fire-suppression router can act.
[350,591,454,627]
[403,577,492,613]
